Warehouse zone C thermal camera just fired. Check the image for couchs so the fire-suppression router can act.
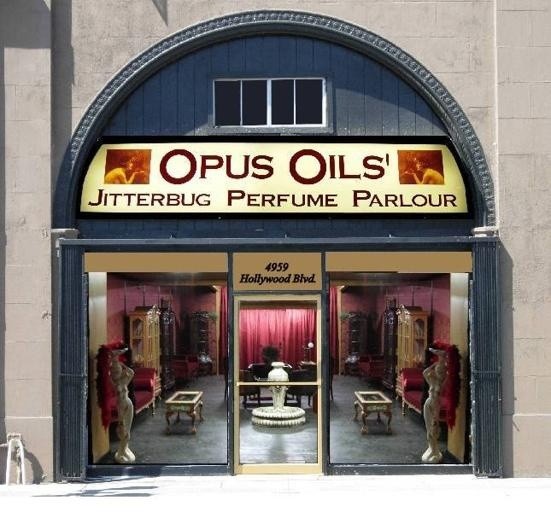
[401,367,448,440]
[247,362,293,388]
[109,366,158,424]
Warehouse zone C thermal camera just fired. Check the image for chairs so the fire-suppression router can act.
[289,368,314,406]
[225,369,261,408]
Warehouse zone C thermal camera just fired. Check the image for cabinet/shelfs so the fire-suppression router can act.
[129,304,212,403]
[344,304,430,402]
